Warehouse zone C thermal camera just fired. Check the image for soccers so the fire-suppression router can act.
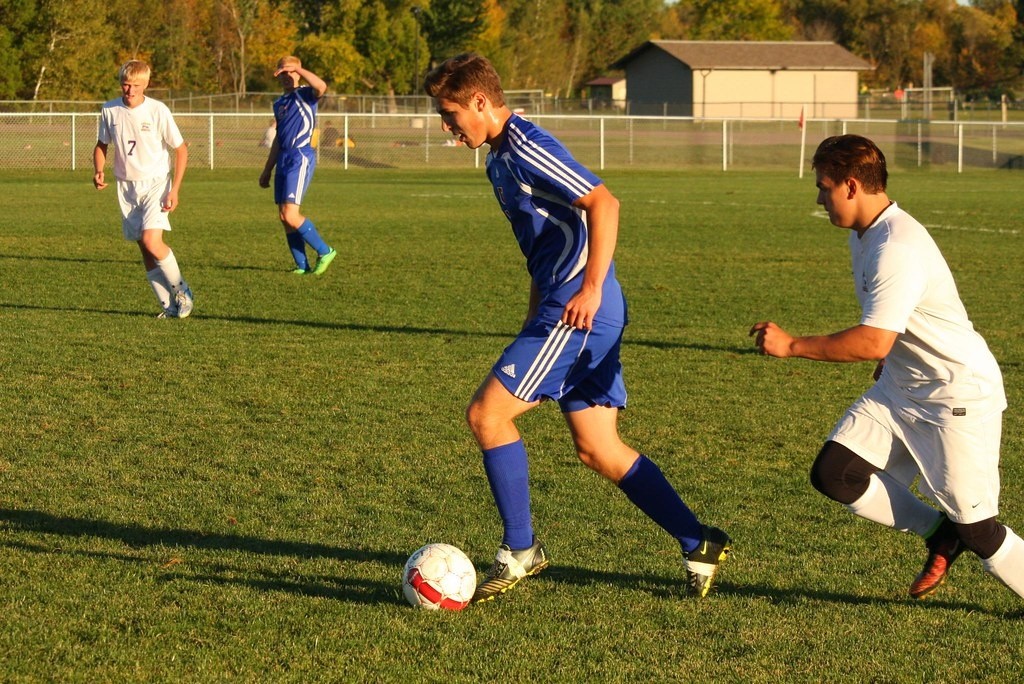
[404,543,477,612]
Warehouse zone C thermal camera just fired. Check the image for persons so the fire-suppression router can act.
[265,120,276,148]
[750,135,1024,600]
[321,121,339,147]
[93,62,195,318]
[422,50,733,605]
[259,56,338,275]
[311,122,320,150]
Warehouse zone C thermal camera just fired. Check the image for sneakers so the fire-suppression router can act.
[313,246,337,276]
[909,512,968,599]
[472,539,551,605]
[156,289,194,320]
[289,268,308,275]
[683,525,731,598]
